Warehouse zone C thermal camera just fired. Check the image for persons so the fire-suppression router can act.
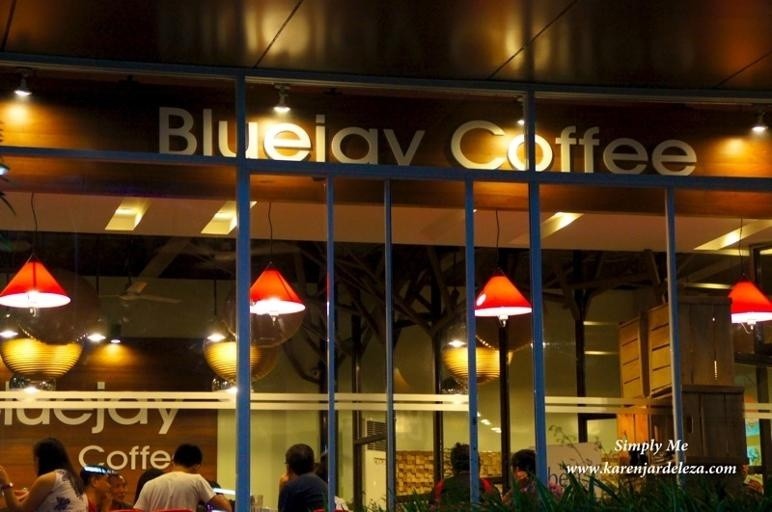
[275,443,337,512]
[107,470,133,511]
[310,463,349,512]
[425,441,502,511]
[502,449,562,504]
[81,464,114,512]
[277,471,295,512]
[0,436,89,512]
[133,466,166,507]
[199,479,223,506]
[131,440,235,512]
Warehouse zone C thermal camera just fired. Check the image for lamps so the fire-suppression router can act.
[728,218,772,330]
[249,200,307,315]
[0,192,71,316]
[473,209,532,326]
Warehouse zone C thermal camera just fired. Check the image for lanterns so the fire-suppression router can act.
[441,325,513,386]
[202,330,283,386]
[1,328,87,379]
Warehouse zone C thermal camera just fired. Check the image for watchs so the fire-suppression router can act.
[1,482,14,490]
